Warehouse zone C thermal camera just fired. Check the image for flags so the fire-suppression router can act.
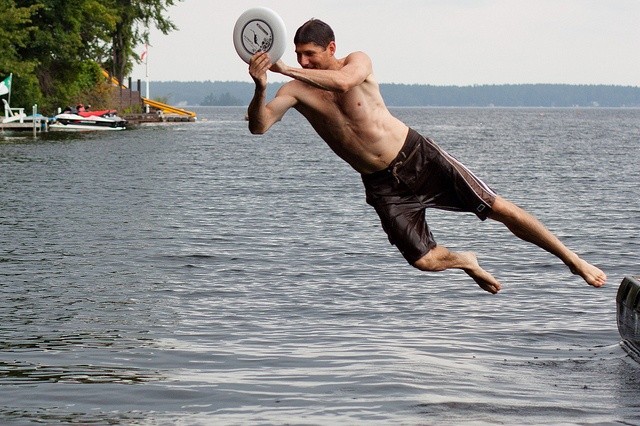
[0,76,11,96]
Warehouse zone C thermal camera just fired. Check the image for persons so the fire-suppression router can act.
[247,19,607,295]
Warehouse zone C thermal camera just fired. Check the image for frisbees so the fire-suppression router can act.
[233,7,288,66]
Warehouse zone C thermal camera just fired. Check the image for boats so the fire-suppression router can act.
[48,107,128,132]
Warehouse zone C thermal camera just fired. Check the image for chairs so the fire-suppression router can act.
[2,98,25,124]
